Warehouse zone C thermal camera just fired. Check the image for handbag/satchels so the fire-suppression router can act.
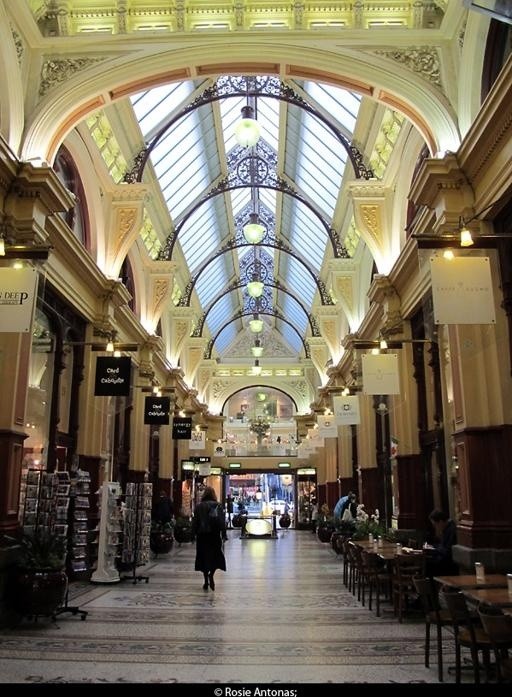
[342,503,353,522]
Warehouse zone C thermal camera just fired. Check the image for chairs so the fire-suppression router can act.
[343,538,433,623]
[425,559,512,684]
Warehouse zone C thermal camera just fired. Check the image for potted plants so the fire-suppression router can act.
[331,517,358,554]
[173,516,195,543]
[149,519,174,554]
[351,519,390,574]
[4,522,73,615]
[316,514,337,542]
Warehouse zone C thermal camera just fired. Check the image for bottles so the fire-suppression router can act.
[373,539,378,550]
[369,533,373,543]
[475,562,485,580]
[506,573,512,595]
[378,535,383,547]
[396,543,402,553]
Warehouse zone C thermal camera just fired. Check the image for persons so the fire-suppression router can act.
[423,508,457,612]
[156,490,175,526]
[334,491,353,532]
[191,486,228,591]
[226,495,235,522]
[341,494,358,522]
[310,499,318,534]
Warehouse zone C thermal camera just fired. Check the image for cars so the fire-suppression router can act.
[234,506,263,518]
[270,500,290,514]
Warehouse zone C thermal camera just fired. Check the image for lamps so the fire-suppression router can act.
[0,232,6,256]
[458,215,473,247]
[378,329,388,349]
[235,76,261,375]
[102,333,114,352]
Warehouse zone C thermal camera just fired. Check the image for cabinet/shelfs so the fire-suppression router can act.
[69,470,91,581]
[90,481,123,582]
